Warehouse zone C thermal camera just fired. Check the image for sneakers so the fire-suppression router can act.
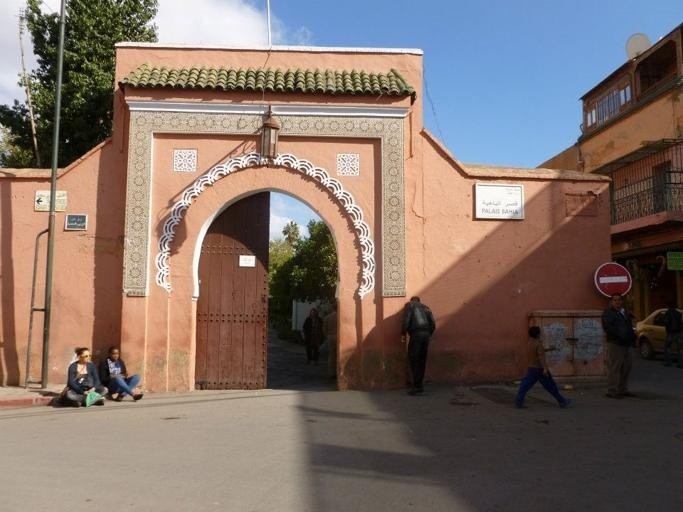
[558,397,572,407]
[71,398,82,407]
[93,398,105,405]
[605,388,636,398]
[132,392,143,400]
[114,393,124,401]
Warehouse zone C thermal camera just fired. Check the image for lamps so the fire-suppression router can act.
[258,104,280,160]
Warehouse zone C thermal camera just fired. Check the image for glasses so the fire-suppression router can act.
[82,353,91,357]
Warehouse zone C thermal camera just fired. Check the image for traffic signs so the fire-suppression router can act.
[34,189,67,212]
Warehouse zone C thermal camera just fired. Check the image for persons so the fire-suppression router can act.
[66,347,109,407]
[664,299,683,368]
[516,326,572,408]
[302,308,324,361]
[99,346,143,401]
[400,297,435,394]
[602,292,638,398]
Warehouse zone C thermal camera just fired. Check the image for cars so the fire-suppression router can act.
[634,306,682,361]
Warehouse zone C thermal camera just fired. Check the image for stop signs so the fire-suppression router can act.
[592,261,633,299]
